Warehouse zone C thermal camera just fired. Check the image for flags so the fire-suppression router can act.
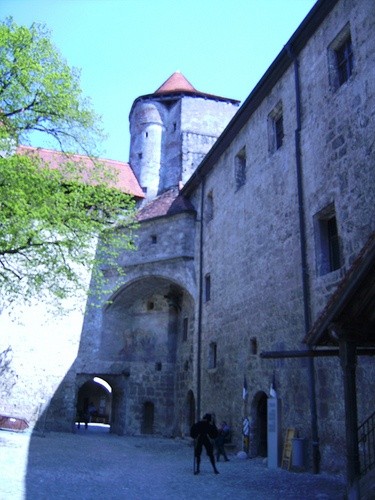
[243,376,248,400]
[269,374,278,399]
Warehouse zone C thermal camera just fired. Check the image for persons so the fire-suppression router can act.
[76,406,91,428]
[191,412,233,475]
